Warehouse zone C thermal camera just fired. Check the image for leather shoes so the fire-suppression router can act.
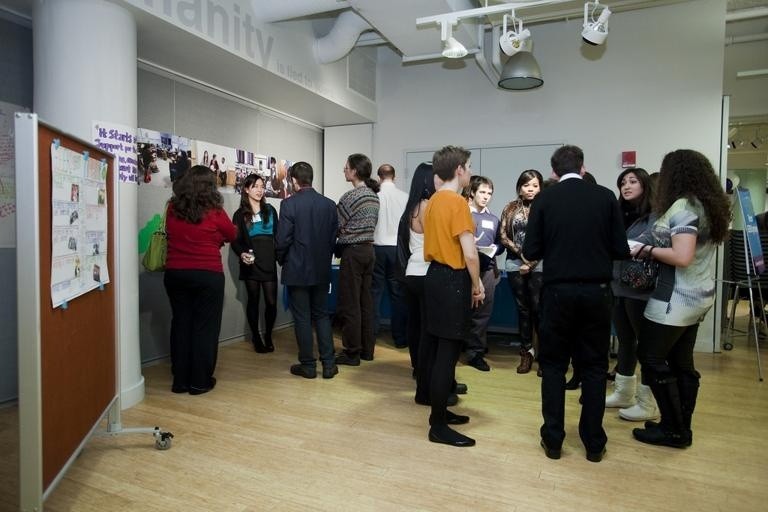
[170,335,374,395]
[412,353,615,462]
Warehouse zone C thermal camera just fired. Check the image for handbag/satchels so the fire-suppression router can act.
[619,260,659,291]
[141,230,167,272]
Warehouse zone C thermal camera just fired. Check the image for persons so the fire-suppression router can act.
[420,143,486,447]
[628,146,732,452]
[459,175,506,372]
[138,139,192,182]
[163,165,239,396]
[230,174,278,353]
[274,161,338,378]
[333,153,468,408]
[496,170,559,376]
[605,168,661,421]
[523,143,632,462]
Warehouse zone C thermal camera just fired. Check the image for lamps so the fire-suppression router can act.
[415,0,612,91]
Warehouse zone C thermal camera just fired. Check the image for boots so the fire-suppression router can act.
[646,383,697,449]
[633,385,689,445]
[617,382,661,420]
[605,369,636,407]
